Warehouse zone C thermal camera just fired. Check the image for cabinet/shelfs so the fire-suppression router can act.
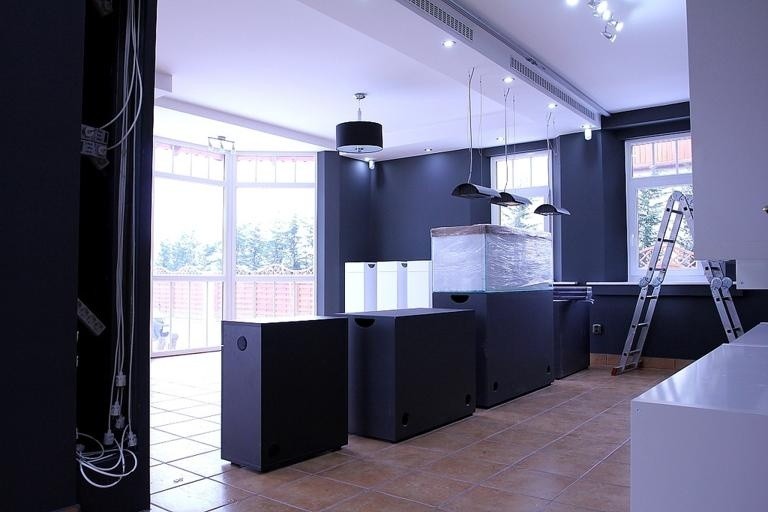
[725,317,767,345]
[370,257,408,313]
[340,299,481,449]
[550,290,593,382]
[405,259,432,309]
[214,314,358,476]
[682,0,767,262]
[427,289,561,416]
[623,342,768,512]
[340,257,376,316]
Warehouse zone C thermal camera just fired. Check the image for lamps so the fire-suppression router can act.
[332,92,389,158]
[568,1,624,46]
[531,104,571,221]
[449,67,501,204]
[490,85,534,211]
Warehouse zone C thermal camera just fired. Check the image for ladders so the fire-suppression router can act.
[611,191,744,375]
